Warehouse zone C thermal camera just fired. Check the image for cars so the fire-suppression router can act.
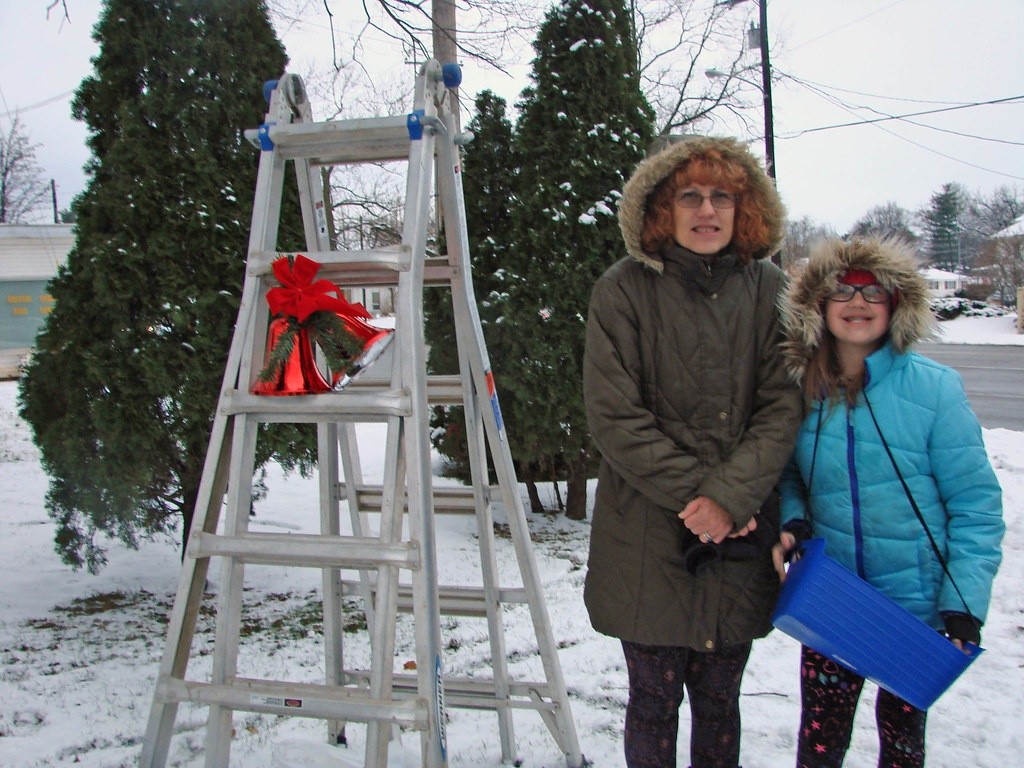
[925,292,1008,322]
[782,257,810,276]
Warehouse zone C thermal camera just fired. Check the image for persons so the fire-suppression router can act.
[582,136,804,768]
[773,233,1006,768]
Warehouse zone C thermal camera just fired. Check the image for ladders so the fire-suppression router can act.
[138,55,586,768]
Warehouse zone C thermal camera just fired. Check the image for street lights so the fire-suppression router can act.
[701,65,784,271]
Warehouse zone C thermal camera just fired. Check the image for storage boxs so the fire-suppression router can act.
[770,536,987,710]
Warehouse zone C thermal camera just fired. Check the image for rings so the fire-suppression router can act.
[704,531,713,541]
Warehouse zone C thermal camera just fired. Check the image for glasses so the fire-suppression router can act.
[827,281,892,304]
[675,189,737,210]
